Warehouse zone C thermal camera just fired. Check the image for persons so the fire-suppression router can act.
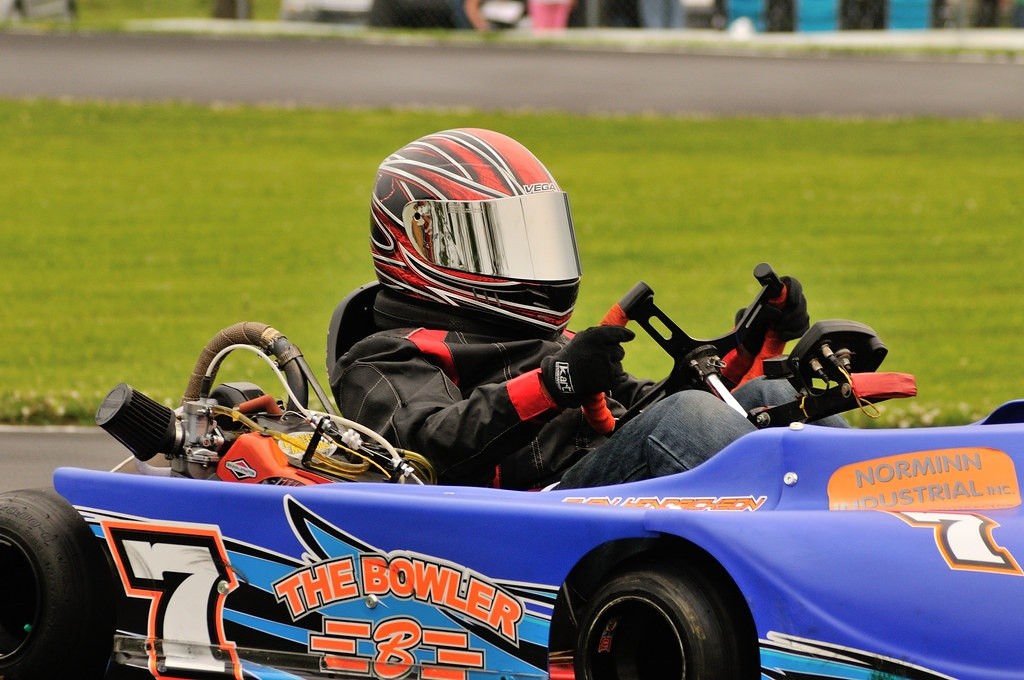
[335,126,861,487]
[359,0,1024,34]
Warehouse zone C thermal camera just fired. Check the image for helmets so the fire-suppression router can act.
[370,127,582,342]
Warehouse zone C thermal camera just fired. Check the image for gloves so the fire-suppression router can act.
[735,277,811,355]
[541,325,634,410]
